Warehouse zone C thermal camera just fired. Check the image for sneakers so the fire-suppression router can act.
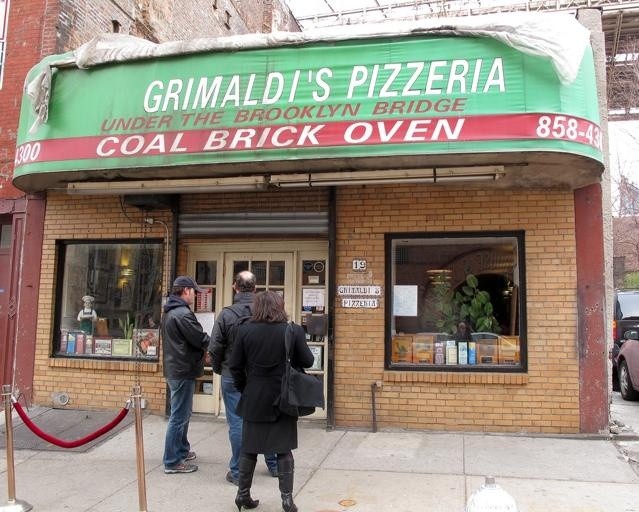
[185,452,195,460]
[226,471,239,486]
[165,460,196,473]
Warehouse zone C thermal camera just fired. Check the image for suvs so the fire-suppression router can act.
[613,287,639,374]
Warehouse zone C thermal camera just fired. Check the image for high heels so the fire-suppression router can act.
[235,488,259,512]
[281,492,297,512]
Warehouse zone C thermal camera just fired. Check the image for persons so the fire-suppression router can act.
[230,290,314,512]
[450,322,471,341]
[207,269,279,486]
[160,276,208,474]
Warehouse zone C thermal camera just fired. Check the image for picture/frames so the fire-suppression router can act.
[87,269,112,303]
[59,327,158,356]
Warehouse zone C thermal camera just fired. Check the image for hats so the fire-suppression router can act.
[173,276,205,292]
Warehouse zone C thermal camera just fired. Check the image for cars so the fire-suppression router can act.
[468,332,518,360]
[615,322,639,404]
[410,333,452,358]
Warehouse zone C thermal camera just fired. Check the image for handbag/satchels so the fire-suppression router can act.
[279,367,324,416]
